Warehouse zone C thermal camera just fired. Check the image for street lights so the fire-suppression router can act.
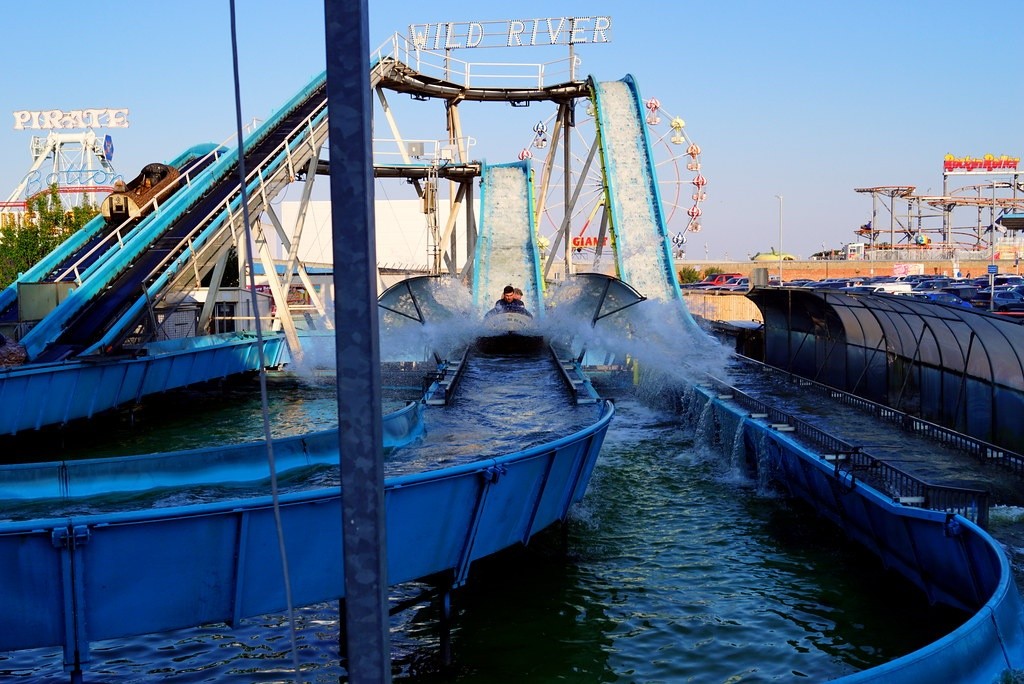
[774,194,784,287]
[986,179,995,310]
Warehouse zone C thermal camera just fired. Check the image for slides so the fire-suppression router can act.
[0,76,1024,684]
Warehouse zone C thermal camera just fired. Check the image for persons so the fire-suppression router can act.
[496,286,525,308]
[867,220,871,228]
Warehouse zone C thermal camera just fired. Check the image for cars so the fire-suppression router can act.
[679,272,1024,313]
